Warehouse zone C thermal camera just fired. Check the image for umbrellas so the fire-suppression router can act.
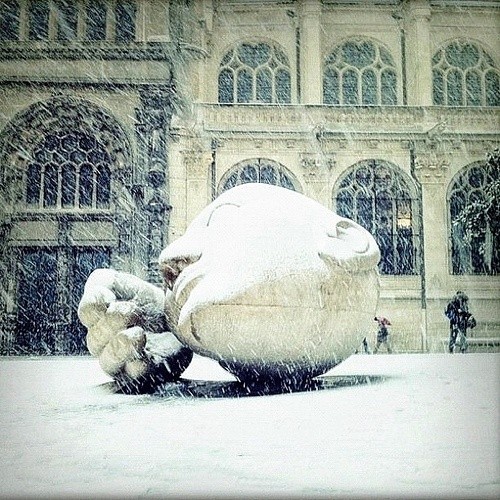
[374,316,391,325]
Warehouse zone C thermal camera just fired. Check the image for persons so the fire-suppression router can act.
[74,182,382,397]
[374,323,391,353]
[444,291,475,352]
